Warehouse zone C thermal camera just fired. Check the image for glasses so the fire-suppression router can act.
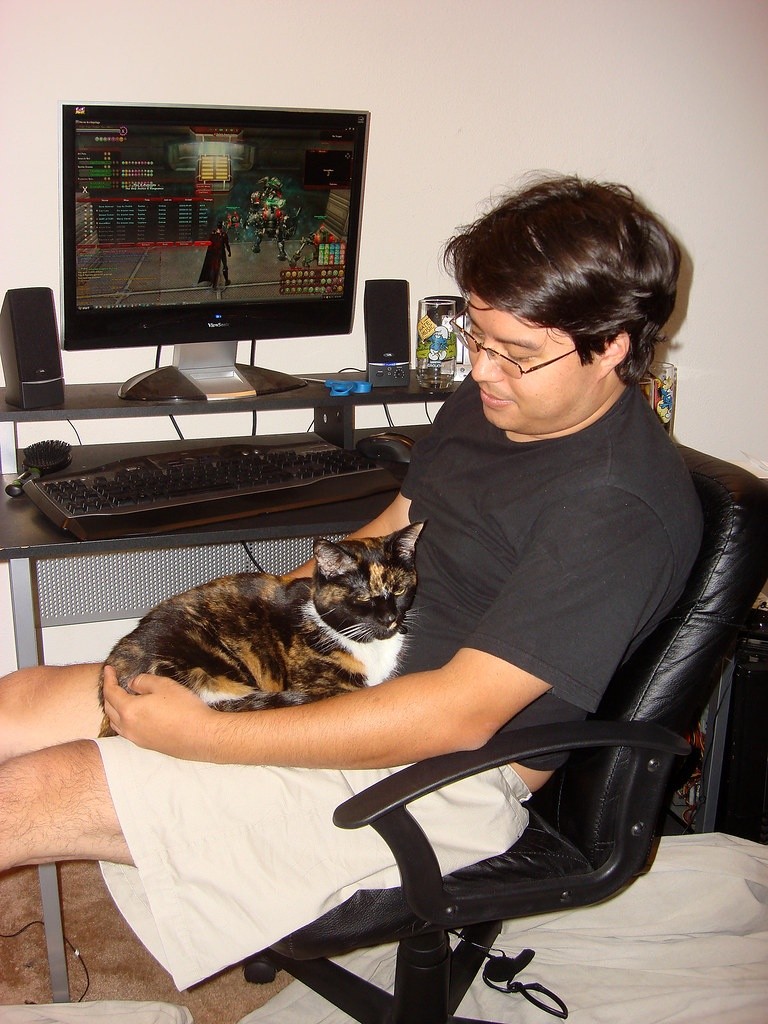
[449,308,600,378]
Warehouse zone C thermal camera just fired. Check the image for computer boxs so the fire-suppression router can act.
[665,607,768,847]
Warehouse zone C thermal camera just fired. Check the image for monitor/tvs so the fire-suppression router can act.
[60,100,371,402]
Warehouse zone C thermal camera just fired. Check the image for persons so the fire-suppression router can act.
[0,176,704,992]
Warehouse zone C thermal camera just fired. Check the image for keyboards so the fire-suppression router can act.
[23,441,403,541]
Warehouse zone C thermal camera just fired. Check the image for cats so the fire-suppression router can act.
[96,518,427,740]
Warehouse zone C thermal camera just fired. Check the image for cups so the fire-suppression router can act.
[416,300,457,389]
[639,377,654,409]
[649,361,675,436]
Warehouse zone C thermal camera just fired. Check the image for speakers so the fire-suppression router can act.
[0,287,65,410]
[363,279,410,388]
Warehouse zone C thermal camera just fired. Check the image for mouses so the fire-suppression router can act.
[357,432,416,463]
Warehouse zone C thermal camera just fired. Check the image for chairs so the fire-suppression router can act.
[242,444,768,1024]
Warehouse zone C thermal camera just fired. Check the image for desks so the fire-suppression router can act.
[0,366,461,1002]
